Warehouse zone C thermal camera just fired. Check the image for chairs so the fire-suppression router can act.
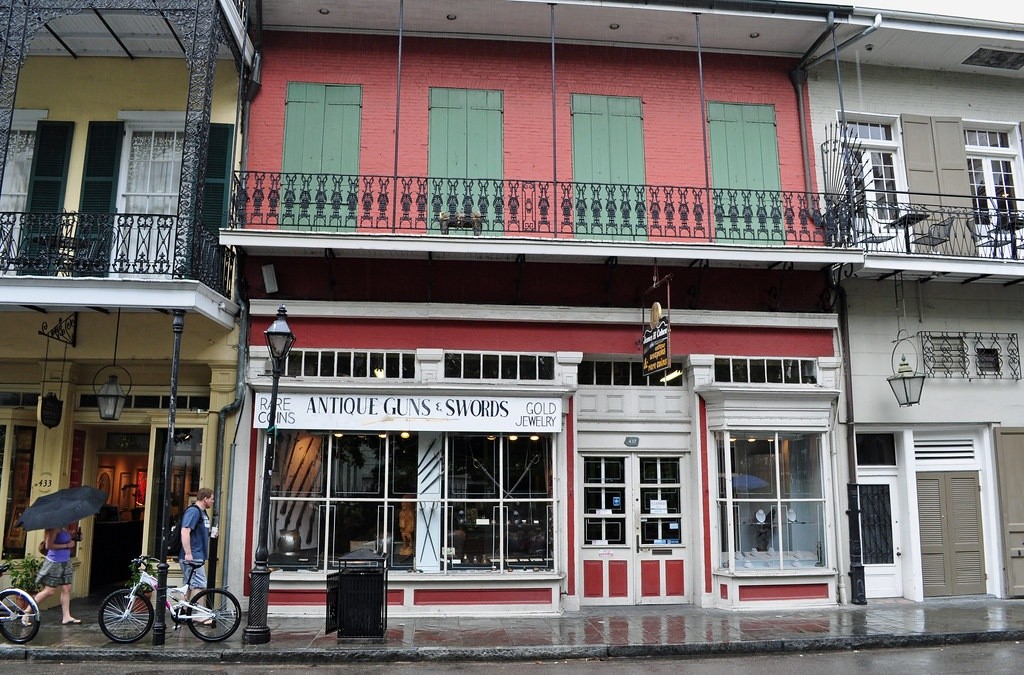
[852,208,898,250]
[0,224,27,276]
[965,216,1012,259]
[80,237,103,277]
[908,216,955,254]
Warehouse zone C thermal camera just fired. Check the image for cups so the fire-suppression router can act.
[210,527,218,538]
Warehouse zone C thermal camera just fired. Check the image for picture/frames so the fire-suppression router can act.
[98,465,116,505]
[173,474,183,505]
[118,472,132,509]
[135,467,148,508]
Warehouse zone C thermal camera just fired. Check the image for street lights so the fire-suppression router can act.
[240,301,298,644]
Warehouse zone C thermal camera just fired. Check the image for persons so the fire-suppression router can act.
[399,495,414,549]
[22,524,82,627]
[178,488,219,626]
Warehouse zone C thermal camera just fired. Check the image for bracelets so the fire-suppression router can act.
[66,542,69,549]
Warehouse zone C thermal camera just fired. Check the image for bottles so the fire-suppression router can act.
[76,527,82,541]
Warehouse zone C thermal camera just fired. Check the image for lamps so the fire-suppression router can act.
[886,336,927,409]
[93,364,134,421]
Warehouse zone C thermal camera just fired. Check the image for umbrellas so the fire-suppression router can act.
[13,485,110,532]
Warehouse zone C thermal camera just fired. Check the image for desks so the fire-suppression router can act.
[28,235,80,277]
[985,221,1024,260]
[884,213,930,253]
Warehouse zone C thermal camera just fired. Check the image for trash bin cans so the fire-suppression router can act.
[323,548,391,644]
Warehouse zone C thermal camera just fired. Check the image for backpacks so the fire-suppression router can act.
[163,505,204,556]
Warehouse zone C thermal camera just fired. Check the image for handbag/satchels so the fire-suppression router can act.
[38,541,48,556]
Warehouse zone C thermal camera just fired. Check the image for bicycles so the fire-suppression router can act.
[98,554,246,641]
[0,564,41,644]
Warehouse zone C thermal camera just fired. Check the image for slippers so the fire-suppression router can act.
[193,617,215,625]
[19,616,32,626]
[61,618,82,625]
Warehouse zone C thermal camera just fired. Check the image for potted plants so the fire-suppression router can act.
[6,554,45,609]
[123,559,155,613]
